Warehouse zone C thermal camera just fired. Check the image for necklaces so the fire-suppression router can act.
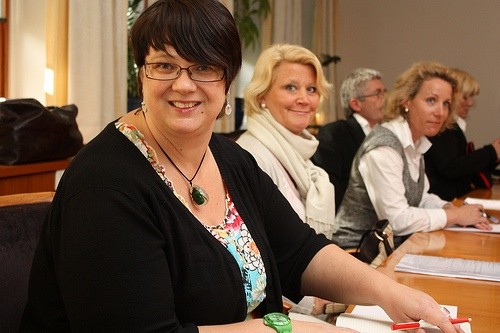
[134,101,210,210]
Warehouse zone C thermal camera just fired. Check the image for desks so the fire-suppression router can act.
[0,156,76,195]
[343,181,500,333]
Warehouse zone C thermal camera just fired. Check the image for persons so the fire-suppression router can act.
[333,65,494,273]
[309,60,500,218]
[234,43,336,240]
[17,0,465,333]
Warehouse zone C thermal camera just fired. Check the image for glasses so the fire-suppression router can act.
[141,61,225,83]
[360,91,386,99]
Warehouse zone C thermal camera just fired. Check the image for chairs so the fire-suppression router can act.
[0,191,56,333]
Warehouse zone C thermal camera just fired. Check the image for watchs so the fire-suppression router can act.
[263,311,293,333]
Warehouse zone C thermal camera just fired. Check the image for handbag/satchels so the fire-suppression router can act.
[357,220,395,267]
[0,98,83,165]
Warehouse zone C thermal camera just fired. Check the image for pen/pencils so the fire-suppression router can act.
[389,318,472,330]
[463,201,498,222]
